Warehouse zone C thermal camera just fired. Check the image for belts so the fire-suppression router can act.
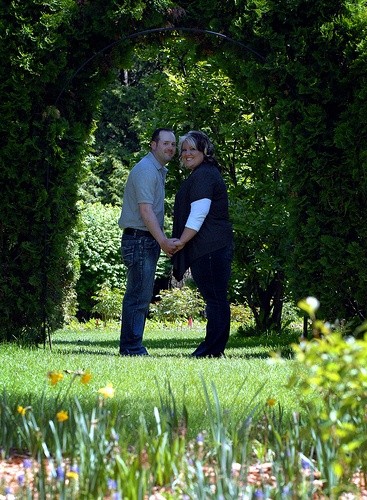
[124,228,152,237]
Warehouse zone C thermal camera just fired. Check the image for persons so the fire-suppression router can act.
[171,131,235,357]
[118,128,180,356]
